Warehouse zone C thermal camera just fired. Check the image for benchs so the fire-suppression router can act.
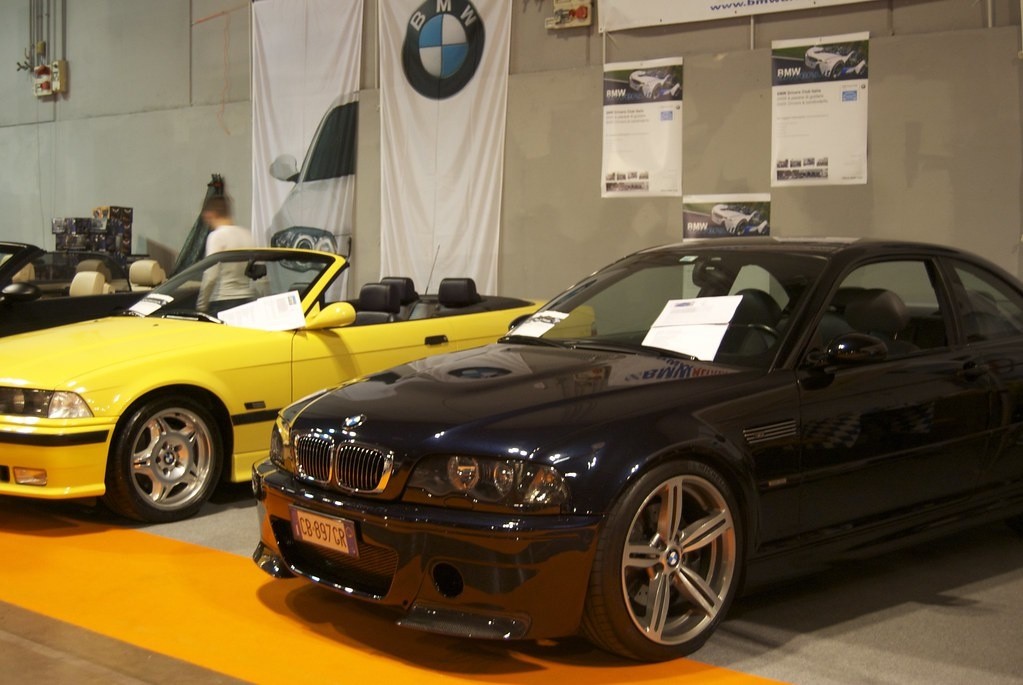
[817,285,1020,348]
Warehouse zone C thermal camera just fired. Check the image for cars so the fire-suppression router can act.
[0,247,596,524]
[0,238,209,339]
[254,233,1022,663]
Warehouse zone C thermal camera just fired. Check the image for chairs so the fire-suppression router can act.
[0,254,36,283]
[845,289,919,355]
[381,276,420,321]
[76,260,113,283]
[724,287,782,353]
[432,277,490,317]
[289,282,326,310]
[69,270,116,297]
[355,283,400,326]
[129,260,166,291]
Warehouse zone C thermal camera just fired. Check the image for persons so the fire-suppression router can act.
[196,199,271,317]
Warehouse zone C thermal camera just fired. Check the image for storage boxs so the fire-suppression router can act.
[52,204,133,254]
